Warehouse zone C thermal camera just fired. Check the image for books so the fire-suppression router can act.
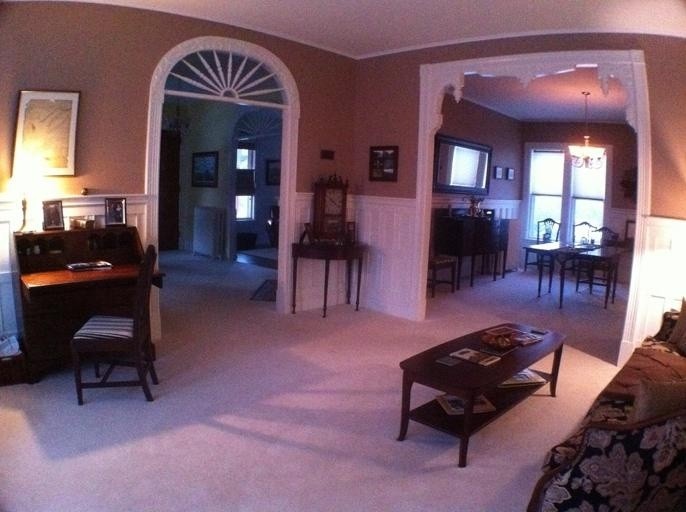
[496,369,546,388]
[435,392,497,416]
[66,261,112,272]
[449,348,501,366]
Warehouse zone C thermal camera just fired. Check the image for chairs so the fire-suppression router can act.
[523,218,562,271]
[561,221,598,282]
[427,231,457,299]
[575,226,620,296]
[481,217,513,278]
[71,244,165,406]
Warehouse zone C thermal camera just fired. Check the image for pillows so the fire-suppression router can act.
[667,298,686,354]
[626,378,686,427]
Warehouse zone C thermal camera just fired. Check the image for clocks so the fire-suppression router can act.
[301,172,351,250]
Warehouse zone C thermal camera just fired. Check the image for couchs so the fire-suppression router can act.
[527,296,686,510]
[267,204,279,249]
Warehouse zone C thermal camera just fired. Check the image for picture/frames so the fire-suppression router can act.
[493,165,503,180]
[42,199,64,231]
[368,145,399,184]
[265,158,280,186]
[506,167,515,181]
[191,150,219,189]
[104,197,127,226]
[299,222,314,244]
[11,89,79,176]
[625,220,636,241]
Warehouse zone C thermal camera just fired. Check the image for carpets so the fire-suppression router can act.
[248,278,278,302]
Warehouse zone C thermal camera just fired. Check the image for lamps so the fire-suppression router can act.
[569,92,606,169]
[2,171,51,235]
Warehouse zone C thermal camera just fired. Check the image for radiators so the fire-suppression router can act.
[191,205,227,260]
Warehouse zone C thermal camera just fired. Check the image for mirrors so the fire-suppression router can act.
[432,133,495,196]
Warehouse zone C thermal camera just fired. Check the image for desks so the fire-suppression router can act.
[18,265,156,383]
[520,241,629,310]
[291,243,367,317]
[433,207,501,291]
